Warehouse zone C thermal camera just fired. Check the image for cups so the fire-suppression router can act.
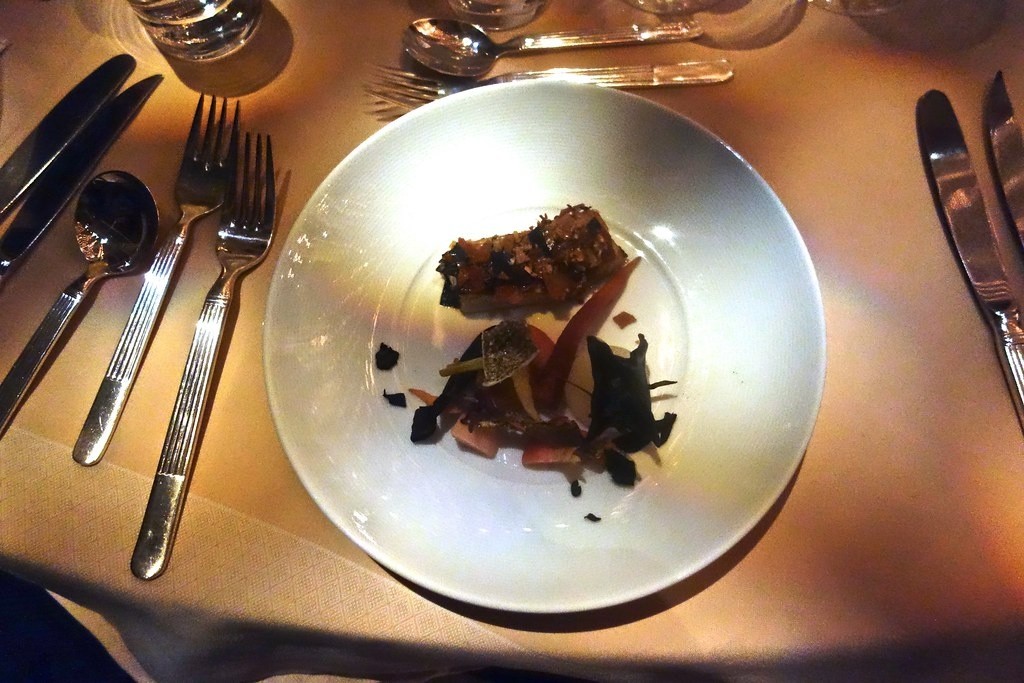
[450,1,540,30]
[129,0,263,63]
[628,1,721,15]
[807,0,907,18]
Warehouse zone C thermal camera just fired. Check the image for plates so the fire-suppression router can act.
[265,78,827,613]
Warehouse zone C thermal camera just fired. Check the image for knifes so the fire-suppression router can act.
[985,70,1024,248]
[0,54,135,216]
[916,90,1024,433]
[0,73,164,279]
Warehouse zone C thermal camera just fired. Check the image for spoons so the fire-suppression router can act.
[0,171,159,438]
[403,17,703,76]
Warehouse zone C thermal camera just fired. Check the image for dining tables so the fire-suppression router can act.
[0,0,1024,683]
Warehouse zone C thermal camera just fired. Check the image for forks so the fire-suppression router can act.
[72,92,240,467]
[364,61,733,110]
[130,132,274,580]
